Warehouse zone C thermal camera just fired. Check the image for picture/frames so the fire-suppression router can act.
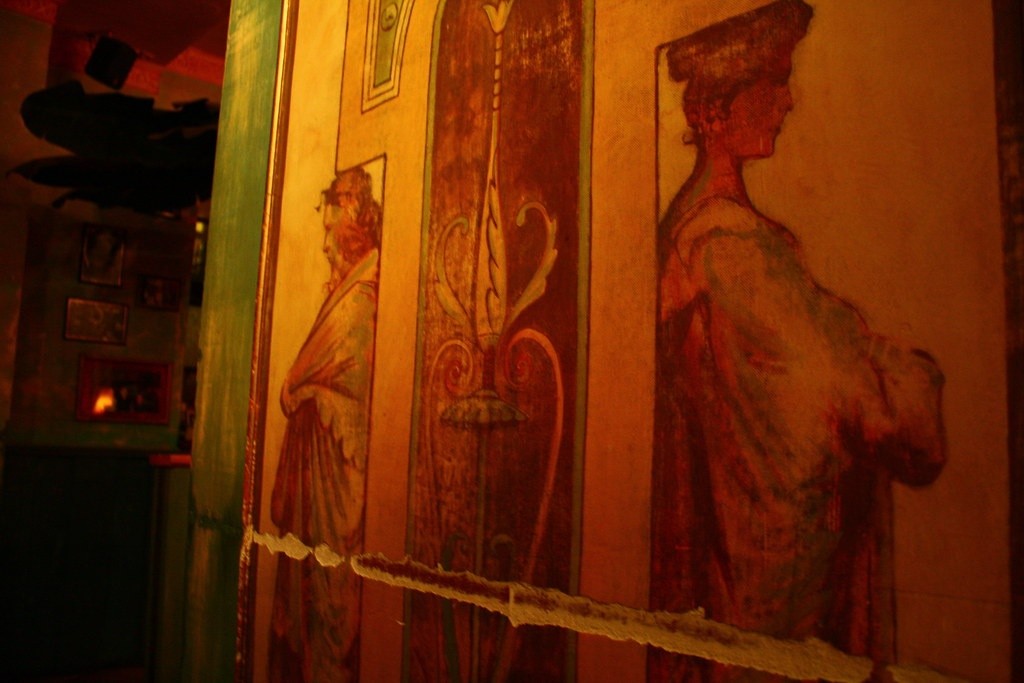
[189,217,209,306]
[74,351,172,425]
[178,368,196,448]
[79,224,127,287]
[64,295,129,343]
[136,272,182,310]
